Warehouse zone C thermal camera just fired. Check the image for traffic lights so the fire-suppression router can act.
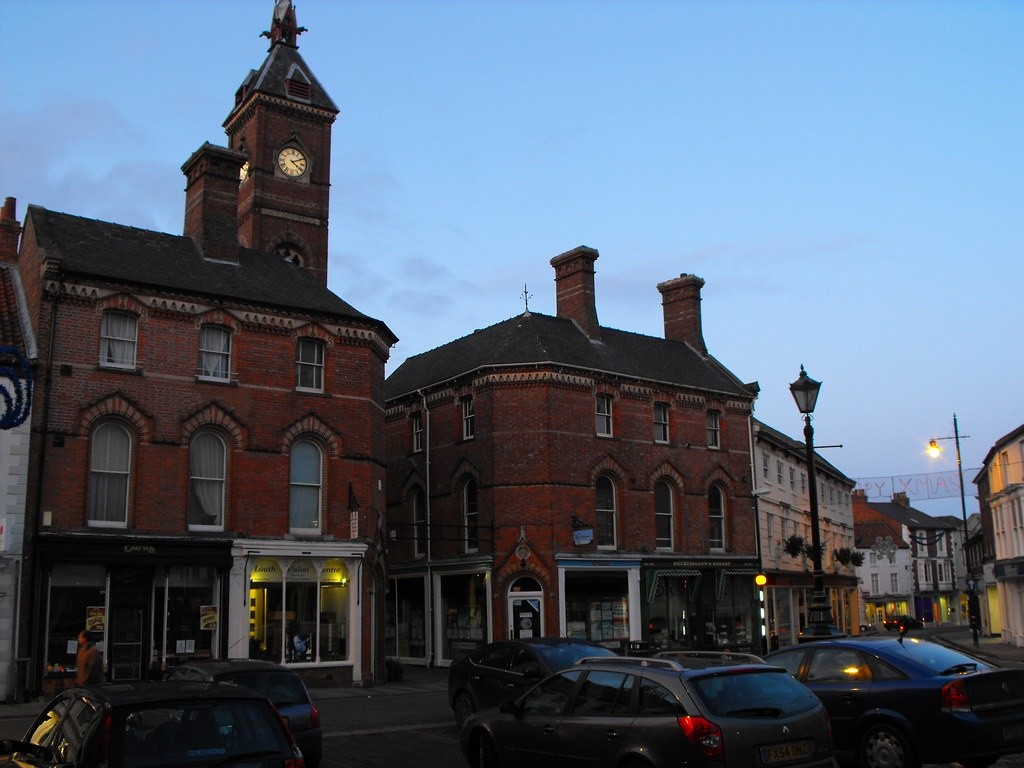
[968,615,980,630]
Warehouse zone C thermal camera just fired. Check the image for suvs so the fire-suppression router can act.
[459,652,838,768]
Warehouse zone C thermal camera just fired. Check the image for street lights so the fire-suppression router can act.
[790,361,847,641]
[924,414,987,640]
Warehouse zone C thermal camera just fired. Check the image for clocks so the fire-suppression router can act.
[278,147,307,177]
[239,160,249,185]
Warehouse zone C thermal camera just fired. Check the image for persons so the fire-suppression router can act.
[67,629,103,688]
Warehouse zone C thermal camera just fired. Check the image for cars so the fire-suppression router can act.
[446,636,634,724]
[883,615,924,630]
[163,658,323,768]
[757,636,1024,768]
[860,623,872,632]
[0,680,305,768]
